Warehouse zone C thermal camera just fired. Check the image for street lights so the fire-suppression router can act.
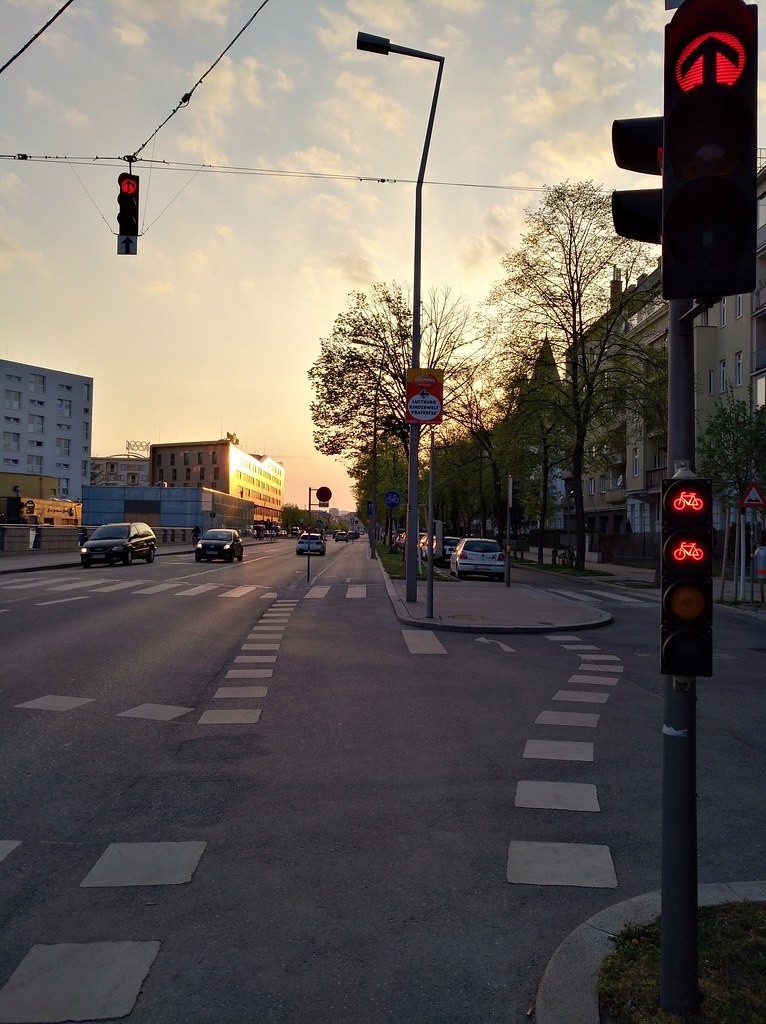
[354,30,448,604]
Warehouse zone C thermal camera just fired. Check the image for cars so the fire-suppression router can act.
[194,528,244,563]
[296,532,326,556]
[332,529,364,543]
[448,537,506,582]
[380,527,462,565]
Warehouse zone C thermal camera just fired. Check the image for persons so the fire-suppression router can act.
[190,525,201,544]
[255,528,267,540]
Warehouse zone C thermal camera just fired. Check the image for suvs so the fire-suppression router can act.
[79,520,158,568]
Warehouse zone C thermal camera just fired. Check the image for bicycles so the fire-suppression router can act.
[558,544,577,568]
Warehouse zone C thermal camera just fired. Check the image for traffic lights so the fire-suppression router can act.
[115,171,140,237]
[610,113,663,245]
[662,1,760,302]
[655,474,718,680]
